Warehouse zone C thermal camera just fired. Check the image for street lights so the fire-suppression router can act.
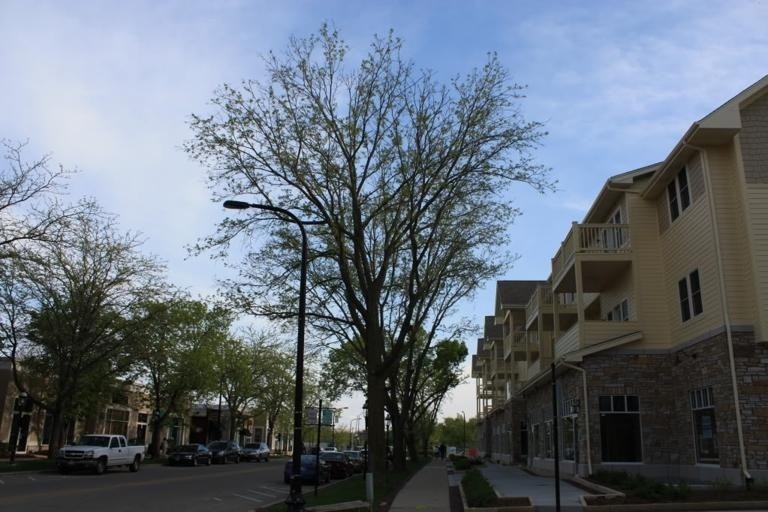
[223,199,307,512]
[331,407,362,450]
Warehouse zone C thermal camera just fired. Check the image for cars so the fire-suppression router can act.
[168,439,271,467]
[284,446,369,485]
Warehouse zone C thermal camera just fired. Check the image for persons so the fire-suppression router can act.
[161,437,168,456]
[439,443,446,460]
[434,446,439,460]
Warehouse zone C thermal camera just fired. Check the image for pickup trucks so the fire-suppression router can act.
[59,433,147,474]
